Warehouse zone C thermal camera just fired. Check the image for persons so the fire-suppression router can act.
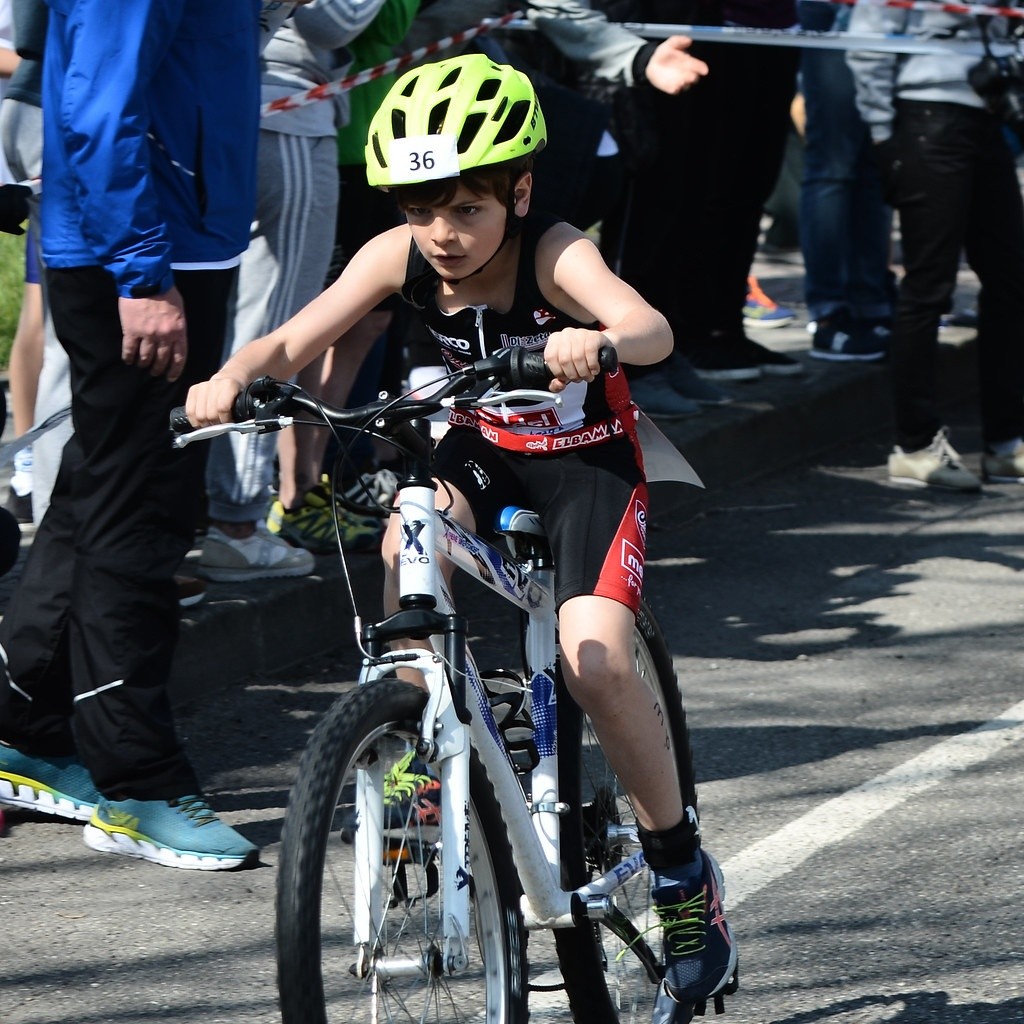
[264,0,419,548]
[0,0,46,525]
[678,0,808,383]
[800,0,898,362]
[0,0,266,873]
[399,1,707,97]
[842,1,1023,493]
[183,52,739,1002]
[31,315,203,604]
[196,0,384,605]
[598,1,735,418]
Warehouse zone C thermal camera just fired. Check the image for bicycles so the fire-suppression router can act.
[166,345,704,1024]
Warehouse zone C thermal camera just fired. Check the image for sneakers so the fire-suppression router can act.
[888,429,985,492]
[809,323,898,363]
[725,337,804,373]
[341,775,447,843]
[332,470,407,513]
[982,447,1024,486]
[0,740,97,821]
[267,475,388,553]
[669,368,737,406]
[650,850,737,1002]
[84,795,260,873]
[687,344,766,380]
[196,523,316,583]
[628,378,701,416]
[738,285,796,328]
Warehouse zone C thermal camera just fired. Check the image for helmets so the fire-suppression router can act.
[366,54,548,194]
